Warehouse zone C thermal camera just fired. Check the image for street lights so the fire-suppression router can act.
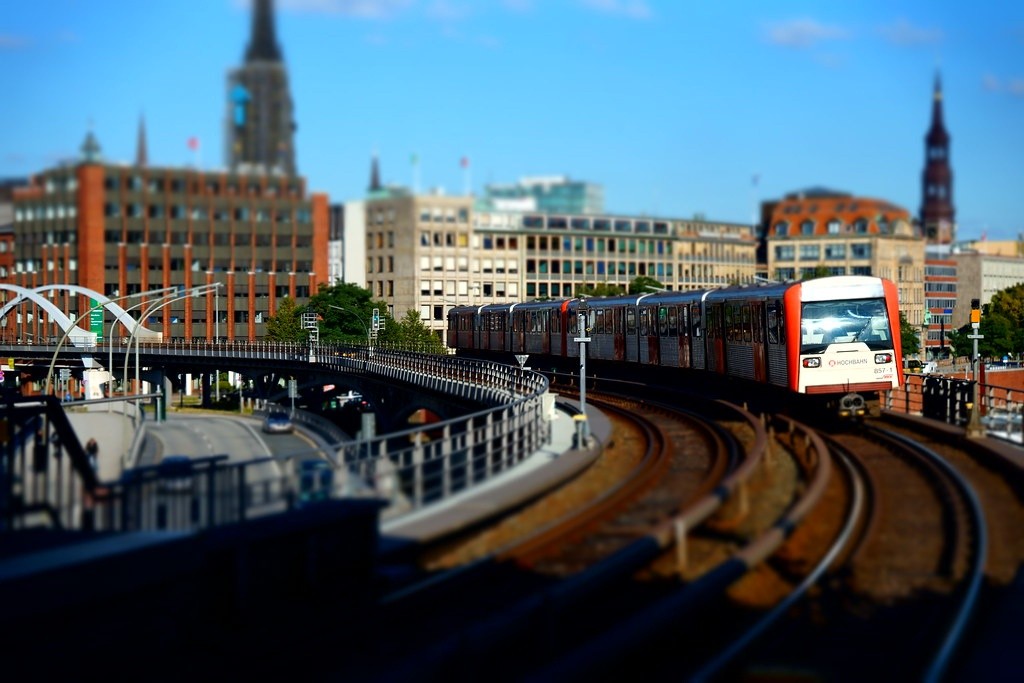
[40,280,224,445]
[331,305,370,357]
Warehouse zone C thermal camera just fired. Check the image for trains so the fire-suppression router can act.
[443,270,905,427]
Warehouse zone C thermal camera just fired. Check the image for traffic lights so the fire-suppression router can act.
[971,298,981,310]
[373,308,380,331]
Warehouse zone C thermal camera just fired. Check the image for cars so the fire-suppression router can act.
[260,409,294,434]
[902,358,937,375]
